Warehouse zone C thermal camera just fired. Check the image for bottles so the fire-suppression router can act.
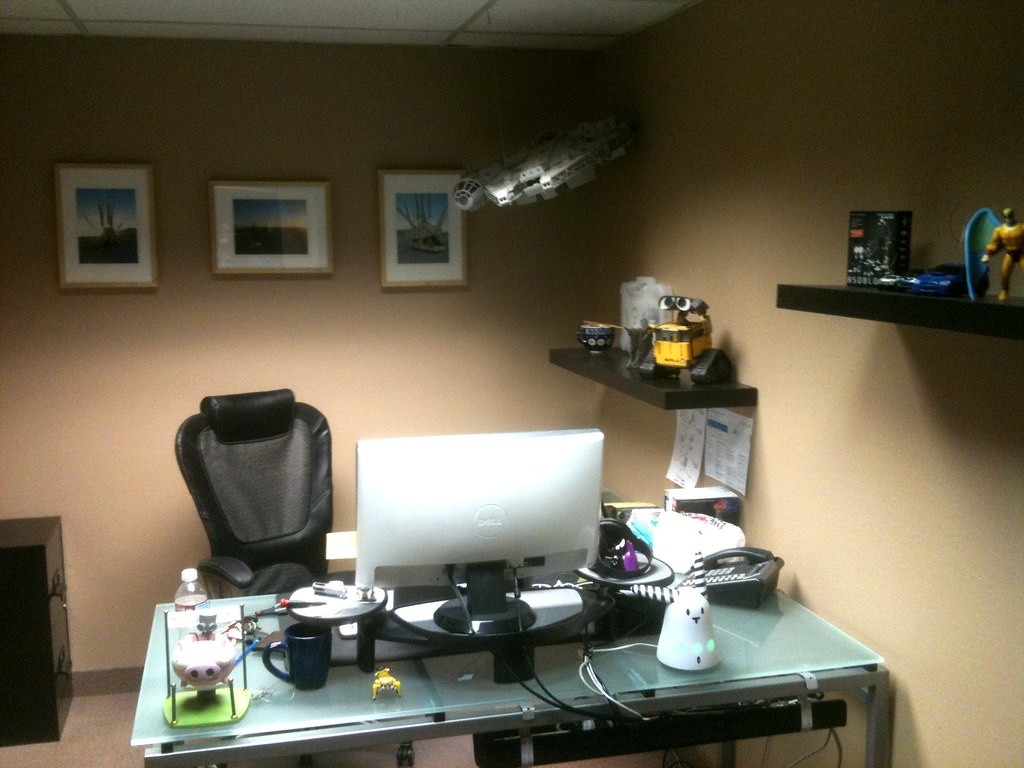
[174,568,208,611]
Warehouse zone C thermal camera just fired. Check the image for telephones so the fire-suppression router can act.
[676,547,784,609]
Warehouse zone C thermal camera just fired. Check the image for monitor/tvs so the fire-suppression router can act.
[355,428,604,637]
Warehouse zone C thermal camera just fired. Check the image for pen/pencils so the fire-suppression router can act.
[234,638,261,667]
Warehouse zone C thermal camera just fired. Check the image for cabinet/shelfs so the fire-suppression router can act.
[0,516,74,746]
[547,283,1024,410]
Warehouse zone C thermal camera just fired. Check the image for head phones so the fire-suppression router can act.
[585,519,654,579]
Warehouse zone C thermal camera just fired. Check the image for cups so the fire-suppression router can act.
[576,324,616,354]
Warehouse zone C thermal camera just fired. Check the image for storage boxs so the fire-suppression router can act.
[664,487,740,526]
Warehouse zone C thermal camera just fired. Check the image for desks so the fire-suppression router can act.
[130,547,891,768]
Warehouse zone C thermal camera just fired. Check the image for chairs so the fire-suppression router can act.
[175,389,445,768]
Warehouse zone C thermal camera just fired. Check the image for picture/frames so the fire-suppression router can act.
[207,180,336,275]
[54,163,158,288]
[378,168,469,287]
